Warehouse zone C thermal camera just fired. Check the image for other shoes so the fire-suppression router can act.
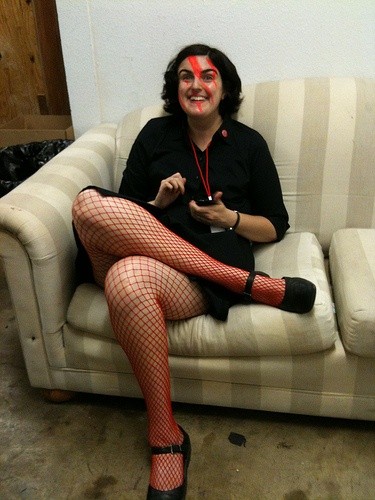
[147,424,192,500]
[243,271,316,314]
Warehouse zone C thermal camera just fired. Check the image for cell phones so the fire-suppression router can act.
[196,199,215,207]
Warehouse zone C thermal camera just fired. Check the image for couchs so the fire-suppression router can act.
[0,76,375,420]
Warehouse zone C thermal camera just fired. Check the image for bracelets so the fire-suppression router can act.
[227,211,240,232]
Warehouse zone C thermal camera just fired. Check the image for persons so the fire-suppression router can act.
[73,43,316,500]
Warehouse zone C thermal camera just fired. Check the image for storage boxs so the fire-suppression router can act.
[0,114,75,148]
